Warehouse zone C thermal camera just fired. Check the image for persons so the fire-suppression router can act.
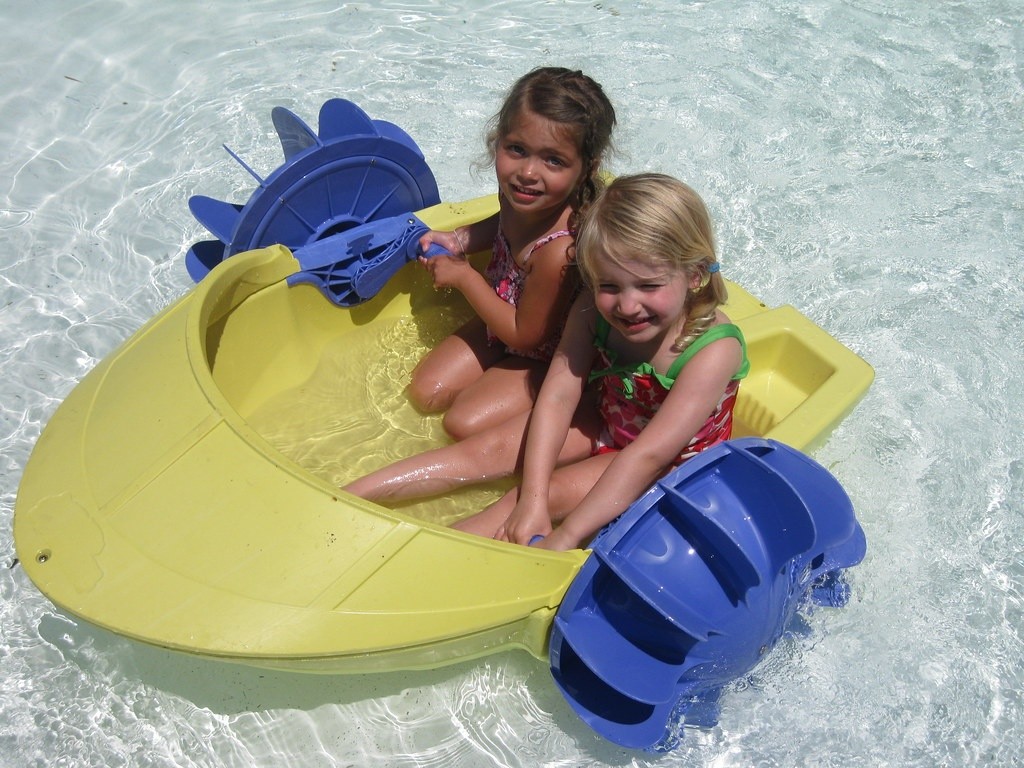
[408,67,617,442]
[339,173,752,553]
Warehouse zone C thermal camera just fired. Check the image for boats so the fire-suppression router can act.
[14,98,876,754]
